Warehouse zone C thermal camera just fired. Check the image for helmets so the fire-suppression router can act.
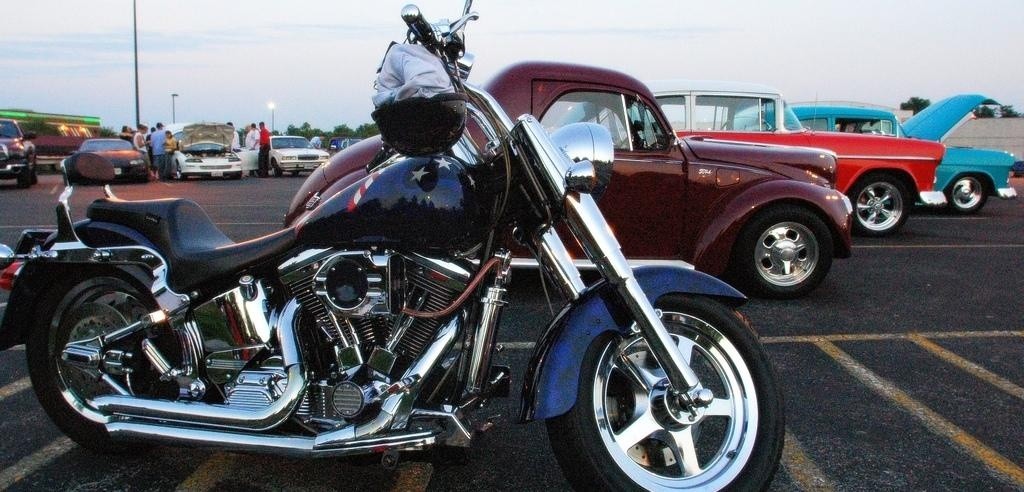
[371,92,469,157]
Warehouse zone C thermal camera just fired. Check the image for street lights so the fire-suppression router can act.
[269,102,274,133]
[169,91,178,122]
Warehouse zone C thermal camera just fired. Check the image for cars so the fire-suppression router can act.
[654,89,947,240]
[278,57,857,300]
[0,116,38,185]
[163,121,260,183]
[733,94,1018,212]
[326,135,364,156]
[253,134,331,177]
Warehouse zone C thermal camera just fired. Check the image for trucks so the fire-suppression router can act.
[0,109,101,173]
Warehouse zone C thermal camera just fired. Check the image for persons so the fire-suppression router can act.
[121,123,177,183]
[228,121,270,179]
[307,135,327,148]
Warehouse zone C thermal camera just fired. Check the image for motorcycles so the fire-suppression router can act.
[0,0,789,492]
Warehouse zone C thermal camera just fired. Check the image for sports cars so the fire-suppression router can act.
[74,135,148,187]
